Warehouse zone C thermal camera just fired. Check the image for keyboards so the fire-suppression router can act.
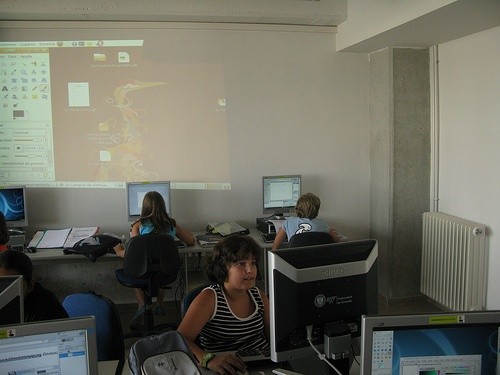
[174,239,186,248]
[7,244,24,253]
[235,348,271,361]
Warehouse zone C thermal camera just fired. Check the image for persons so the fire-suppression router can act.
[0,212,70,324]
[272,192,341,251]
[114,192,197,331]
[178,234,270,375]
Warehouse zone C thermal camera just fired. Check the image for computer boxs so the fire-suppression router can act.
[256,216,290,235]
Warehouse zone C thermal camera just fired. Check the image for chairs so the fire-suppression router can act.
[180,283,212,320]
[49,292,125,361]
[115,234,180,349]
[288,232,336,248]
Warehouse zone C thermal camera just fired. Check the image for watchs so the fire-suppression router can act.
[199,352,216,369]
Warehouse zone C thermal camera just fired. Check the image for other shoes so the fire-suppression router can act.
[129,305,152,331]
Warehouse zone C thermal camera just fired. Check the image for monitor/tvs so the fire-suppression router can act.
[268,239,378,375]
[0,315,99,375]
[126,181,171,222]
[262,174,305,222]
[0,185,28,233]
[360,310,500,375]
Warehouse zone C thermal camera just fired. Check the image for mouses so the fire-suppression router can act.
[236,369,249,375]
[28,247,36,253]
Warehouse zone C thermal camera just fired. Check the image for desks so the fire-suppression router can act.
[26,227,349,294]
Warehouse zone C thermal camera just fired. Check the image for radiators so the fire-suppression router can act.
[420,211,488,312]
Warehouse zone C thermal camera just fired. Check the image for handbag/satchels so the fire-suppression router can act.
[127,331,205,375]
[64,234,122,262]
